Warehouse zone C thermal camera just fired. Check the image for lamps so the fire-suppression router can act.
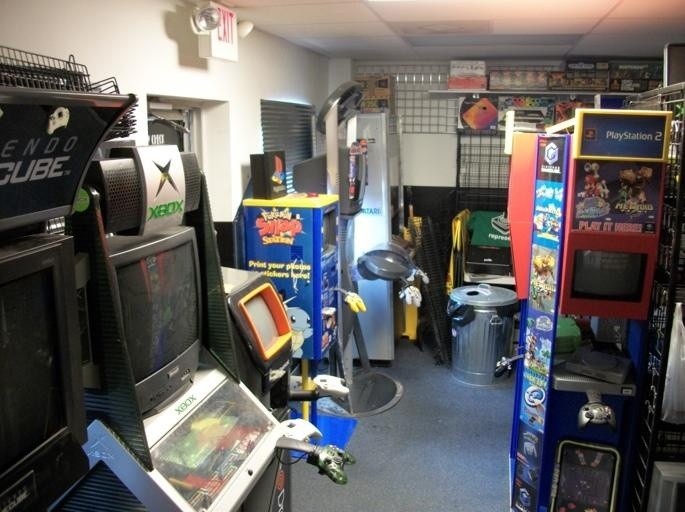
[190,0,254,39]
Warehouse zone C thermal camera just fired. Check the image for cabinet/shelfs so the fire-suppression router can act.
[340,112,404,368]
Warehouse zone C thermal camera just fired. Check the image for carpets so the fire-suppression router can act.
[289,411,358,459]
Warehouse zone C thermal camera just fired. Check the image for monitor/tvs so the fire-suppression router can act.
[0,233,88,512]
[560,231,658,320]
[227,273,293,375]
[293,147,368,215]
[104,225,202,421]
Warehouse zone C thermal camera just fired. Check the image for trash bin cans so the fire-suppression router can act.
[449,283,518,387]
